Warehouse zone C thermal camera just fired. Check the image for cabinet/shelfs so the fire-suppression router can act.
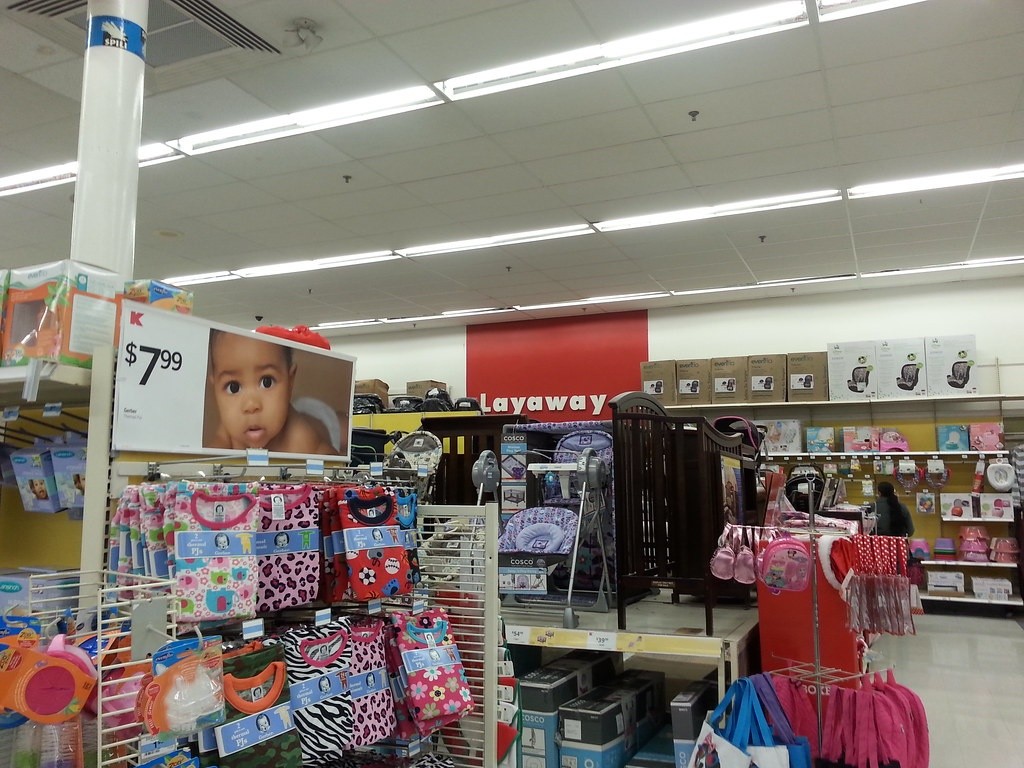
[663,392,1024,607]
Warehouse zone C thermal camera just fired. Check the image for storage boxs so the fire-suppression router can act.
[740,416,1017,601]
[640,333,979,402]
[355,378,389,408]
[519,652,718,768]
[0,259,195,637]
[406,380,446,400]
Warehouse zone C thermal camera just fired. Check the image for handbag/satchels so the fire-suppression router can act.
[684,519,932,768]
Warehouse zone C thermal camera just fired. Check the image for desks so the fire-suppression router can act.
[499,583,758,731]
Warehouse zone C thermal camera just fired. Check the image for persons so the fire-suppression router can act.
[217,534,227,549]
[405,533,409,543]
[31,479,48,499]
[277,533,287,548]
[373,529,381,540]
[217,506,222,513]
[255,688,261,698]
[76,474,85,495]
[369,510,374,516]
[430,650,440,663]
[320,677,330,692]
[867,481,914,536]
[367,673,375,687]
[206,328,348,456]
[321,646,327,653]
[275,496,281,504]
[258,715,269,732]
